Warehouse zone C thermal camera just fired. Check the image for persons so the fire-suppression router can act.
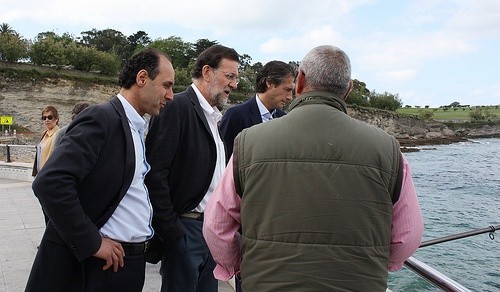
[145,44,238,292]
[202,45,424,292]
[32,106,60,249]
[218,61,296,169]
[24,49,174,292]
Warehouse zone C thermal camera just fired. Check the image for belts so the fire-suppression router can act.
[120,240,151,255]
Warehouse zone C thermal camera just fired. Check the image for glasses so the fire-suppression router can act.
[40,115,54,121]
[216,68,241,84]
